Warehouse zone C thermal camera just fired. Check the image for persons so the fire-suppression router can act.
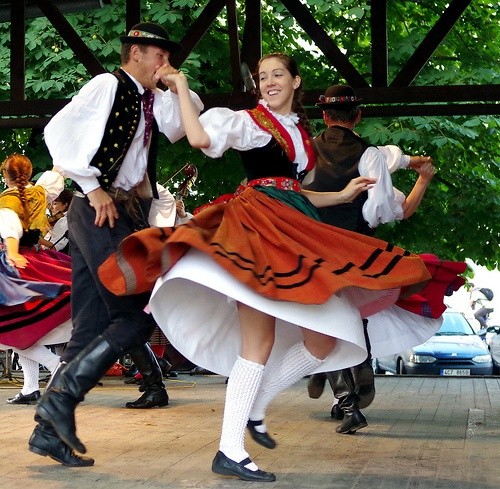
[39,188,74,255]
[469,286,494,330]
[308,160,435,421]
[28,21,205,468]
[299,84,435,434]
[0,153,74,405]
[121,182,219,409]
[97,52,433,482]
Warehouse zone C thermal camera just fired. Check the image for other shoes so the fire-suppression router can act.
[211,451,277,481]
[306,369,326,400]
[125,377,144,384]
[247,418,276,449]
[330,403,346,420]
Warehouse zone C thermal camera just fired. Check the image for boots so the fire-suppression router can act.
[326,370,368,434]
[34,335,121,453]
[353,318,377,409]
[28,422,96,467]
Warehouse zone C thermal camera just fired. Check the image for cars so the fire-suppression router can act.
[371,308,493,376]
[487,325,500,369]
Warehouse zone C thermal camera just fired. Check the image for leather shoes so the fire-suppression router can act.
[139,360,171,392]
[126,388,169,408]
[7,390,41,405]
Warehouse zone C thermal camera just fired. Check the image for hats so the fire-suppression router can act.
[119,22,180,54]
[317,85,362,109]
